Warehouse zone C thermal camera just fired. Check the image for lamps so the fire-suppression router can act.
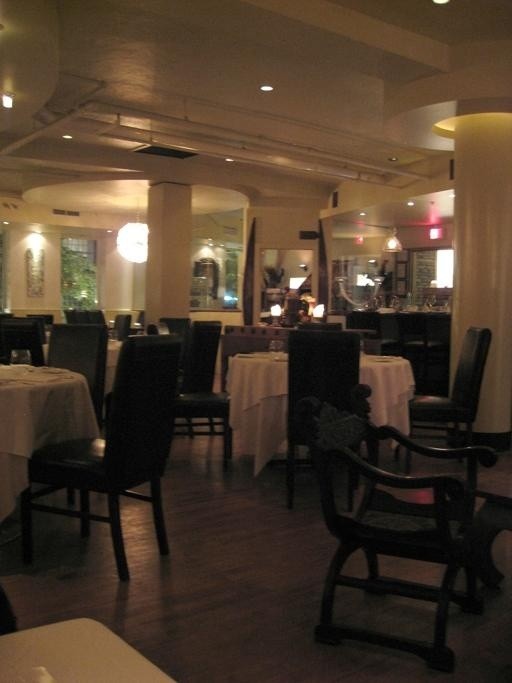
[382,226,402,252]
[116,198,149,263]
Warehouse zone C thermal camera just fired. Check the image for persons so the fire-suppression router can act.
[271,287,296,316]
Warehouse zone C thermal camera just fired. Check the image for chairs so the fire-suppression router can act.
[408,325,490,458]
[293,385,499,673]
[287,312,453,397]
[0,582,18,635]
[0,310,226,443]
[19,333,182,580]
[286,327,362,479]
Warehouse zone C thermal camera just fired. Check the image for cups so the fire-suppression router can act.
[11,349,30,374]
[270,340,285,359]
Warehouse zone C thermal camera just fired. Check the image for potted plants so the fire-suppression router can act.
[262,267,284,301]
